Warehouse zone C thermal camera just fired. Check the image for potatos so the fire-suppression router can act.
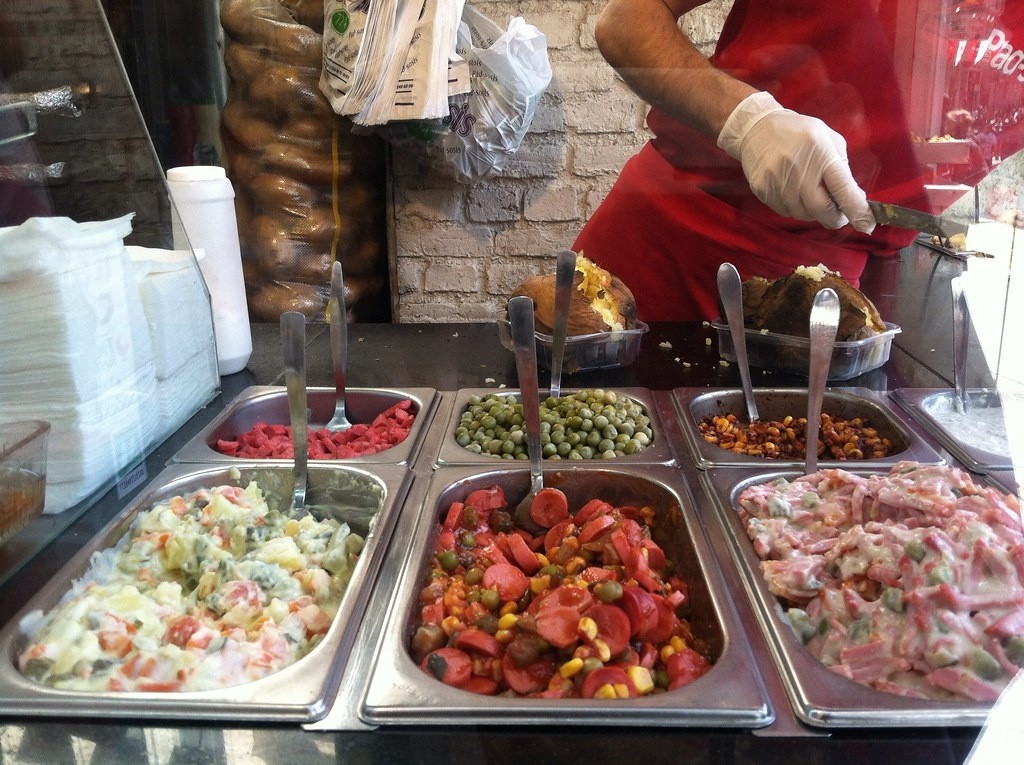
[507,255,637,365]
[219,0,386,321]
[715,264,889,376]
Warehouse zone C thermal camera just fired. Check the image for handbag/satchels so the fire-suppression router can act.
[371,4,554,184]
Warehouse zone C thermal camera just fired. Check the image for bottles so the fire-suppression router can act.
[165,165,253,377]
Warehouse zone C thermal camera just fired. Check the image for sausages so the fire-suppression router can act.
[419,485,710,698]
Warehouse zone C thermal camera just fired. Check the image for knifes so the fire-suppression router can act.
[827,192,968,241]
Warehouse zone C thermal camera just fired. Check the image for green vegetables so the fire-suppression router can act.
[26,482,363,688]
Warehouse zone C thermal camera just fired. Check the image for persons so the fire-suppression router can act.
[571,0,1024,324]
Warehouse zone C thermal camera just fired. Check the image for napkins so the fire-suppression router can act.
[0,212,217,515]
